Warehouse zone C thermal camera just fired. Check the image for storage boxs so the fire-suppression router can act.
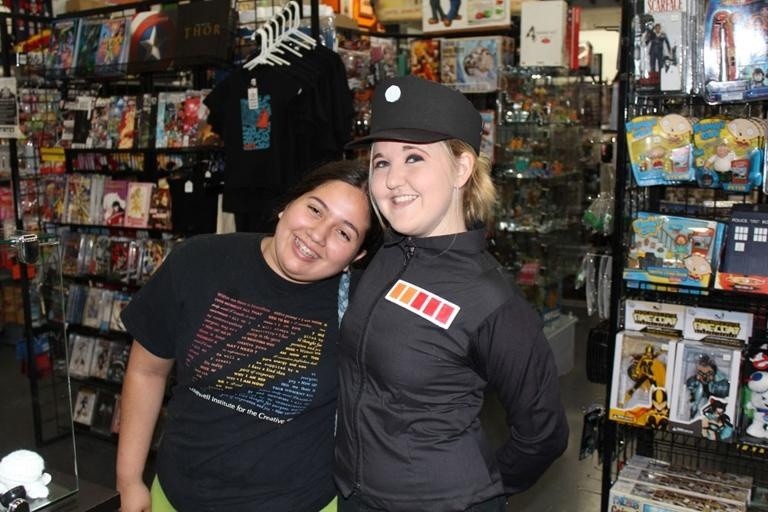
[410,0,580,92]
[542,312,578,377]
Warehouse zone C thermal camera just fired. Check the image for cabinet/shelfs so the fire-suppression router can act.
[600,0,768,512]
[0,1,239,448]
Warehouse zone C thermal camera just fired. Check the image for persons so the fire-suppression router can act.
[99,135,375,512]
[332,77,569,512]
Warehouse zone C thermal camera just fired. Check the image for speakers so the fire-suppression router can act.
[586,319,610,384]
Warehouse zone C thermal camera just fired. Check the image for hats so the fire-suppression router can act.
[343,76,481,157]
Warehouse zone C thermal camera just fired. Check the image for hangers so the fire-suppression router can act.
[243,1,318,72]
